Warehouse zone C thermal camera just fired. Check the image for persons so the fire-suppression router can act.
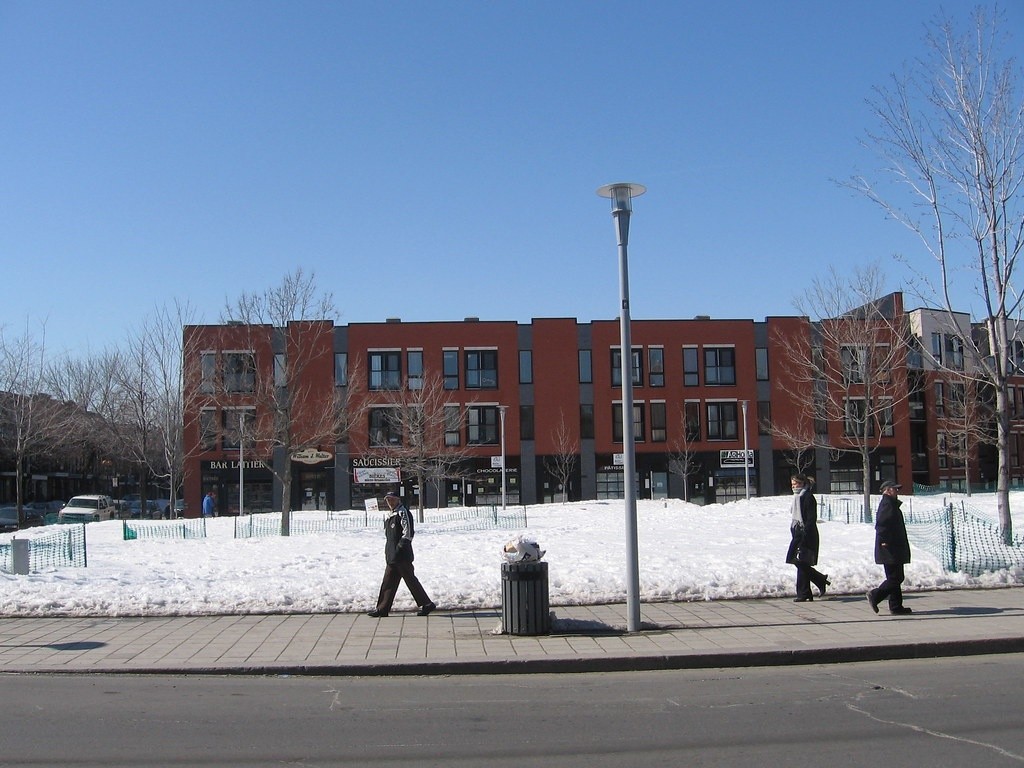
[866,480,912,615]
[203,491,216,517]
[366,487,436,617]
[786,474,831,602]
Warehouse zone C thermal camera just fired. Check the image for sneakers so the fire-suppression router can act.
[417,601,436,616]
[367,608,388,617]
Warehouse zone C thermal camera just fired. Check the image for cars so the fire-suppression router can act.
[114,496,184,520]
[0,506,45,534]
[27,500,67,526]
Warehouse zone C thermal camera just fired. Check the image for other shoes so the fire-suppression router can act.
[891,607,912,614]
[866,591,880,613]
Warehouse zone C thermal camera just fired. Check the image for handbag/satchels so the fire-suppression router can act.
[789,530,814,567]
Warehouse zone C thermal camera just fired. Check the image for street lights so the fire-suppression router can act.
[235,411,249,515]
[595,181,646,634]
[738,400,751,498]
[496,405,510,510]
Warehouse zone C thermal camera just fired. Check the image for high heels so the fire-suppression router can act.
[818,579,831,597]
[794,596,813,601]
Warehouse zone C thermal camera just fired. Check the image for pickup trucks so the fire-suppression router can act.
[58,494,115,524]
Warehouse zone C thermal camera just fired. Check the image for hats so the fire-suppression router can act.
[880,481,903,492]
[384,490,400,501]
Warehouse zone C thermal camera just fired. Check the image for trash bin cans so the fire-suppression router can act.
[500,562,549,635]
